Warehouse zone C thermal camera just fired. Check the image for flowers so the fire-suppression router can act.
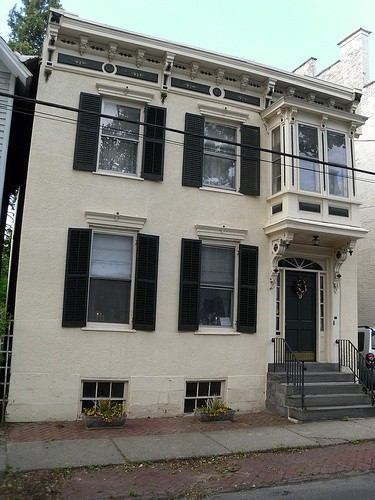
[195,395,229,417]
[83,398,129,423]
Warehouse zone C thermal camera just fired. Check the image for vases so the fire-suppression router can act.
[193,407,235,422]
[83,414,127,430]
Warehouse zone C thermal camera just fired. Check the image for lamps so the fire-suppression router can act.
[311,235,320,246]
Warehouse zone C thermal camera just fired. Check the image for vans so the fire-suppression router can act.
[358,325,375,370]
